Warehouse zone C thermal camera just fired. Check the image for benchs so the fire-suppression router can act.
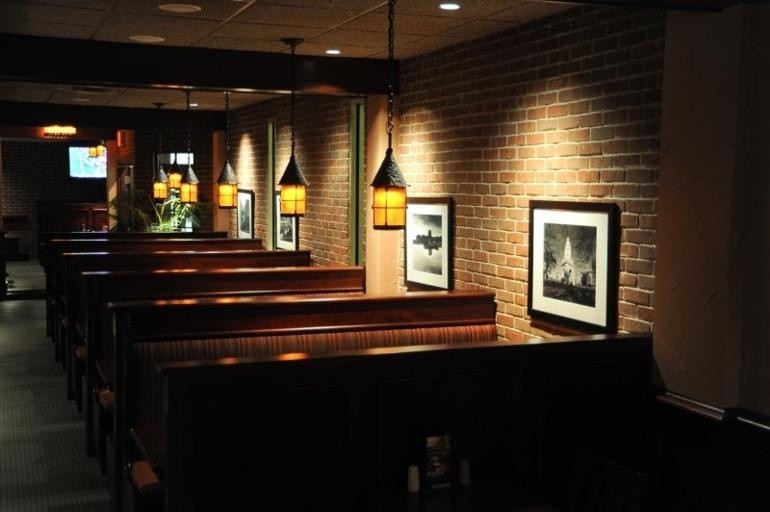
[38,230,662,511]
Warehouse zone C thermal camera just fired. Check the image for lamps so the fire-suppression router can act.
[370,0,413,230]
[180,87,199,203]
[167,114,183,189]
[150,100,167,200]
[279,38,310,217]
[217,90,239,209]
[89,140,106,158]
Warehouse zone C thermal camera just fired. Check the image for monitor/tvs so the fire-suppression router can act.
[66,144,109,181]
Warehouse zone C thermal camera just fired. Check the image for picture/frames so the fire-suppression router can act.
[237,189,255,238]
[527,199,619,335]
[274,190,299,251]
[399,197,455,291]
